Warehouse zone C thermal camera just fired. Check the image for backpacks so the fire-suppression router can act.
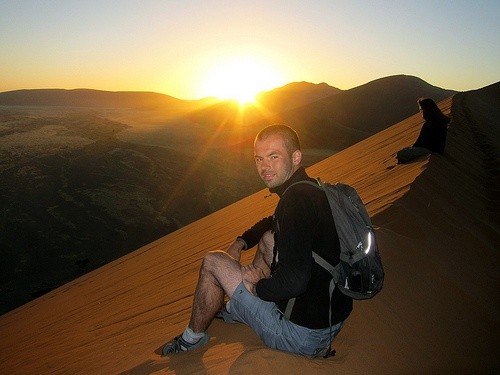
[271,177,384,359]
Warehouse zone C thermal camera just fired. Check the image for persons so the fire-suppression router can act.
[162,124,354,357]
[412,99,450,154]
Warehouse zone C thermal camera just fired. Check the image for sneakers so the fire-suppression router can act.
[215,303,235,323]
[162,330,209,355]
